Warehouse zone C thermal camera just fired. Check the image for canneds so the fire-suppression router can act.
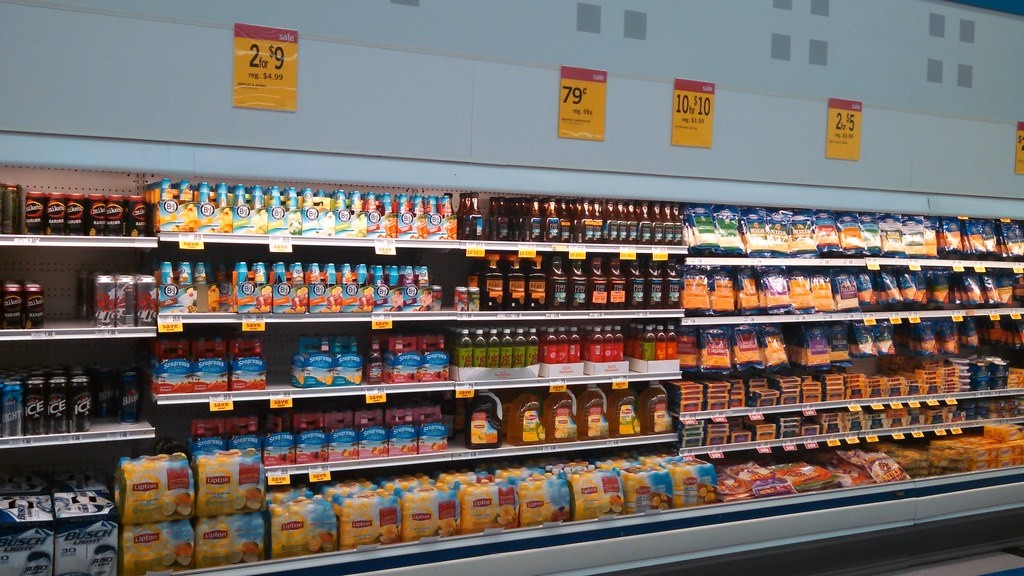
[1,183,156,437]
[455,286,480,312]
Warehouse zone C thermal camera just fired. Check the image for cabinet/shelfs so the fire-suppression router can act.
[0,0,1023,576]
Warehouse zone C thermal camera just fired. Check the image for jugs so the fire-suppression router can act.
[466,380,672,448]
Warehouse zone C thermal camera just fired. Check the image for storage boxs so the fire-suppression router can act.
[670,356,1023,472]
[0,490,116,576]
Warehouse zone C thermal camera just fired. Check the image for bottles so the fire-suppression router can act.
[191,405,445,441]
[441,393,456,440]
[390,332,446,353]
[158,336,262,361]
[539,460,612,477]
[366,337,384,384]
[457,192,683,245]
[447,323,677,369]
[302,332,356,352]
[143,178,455,213]
[163,262,429,287]
[463,251,681,311]
